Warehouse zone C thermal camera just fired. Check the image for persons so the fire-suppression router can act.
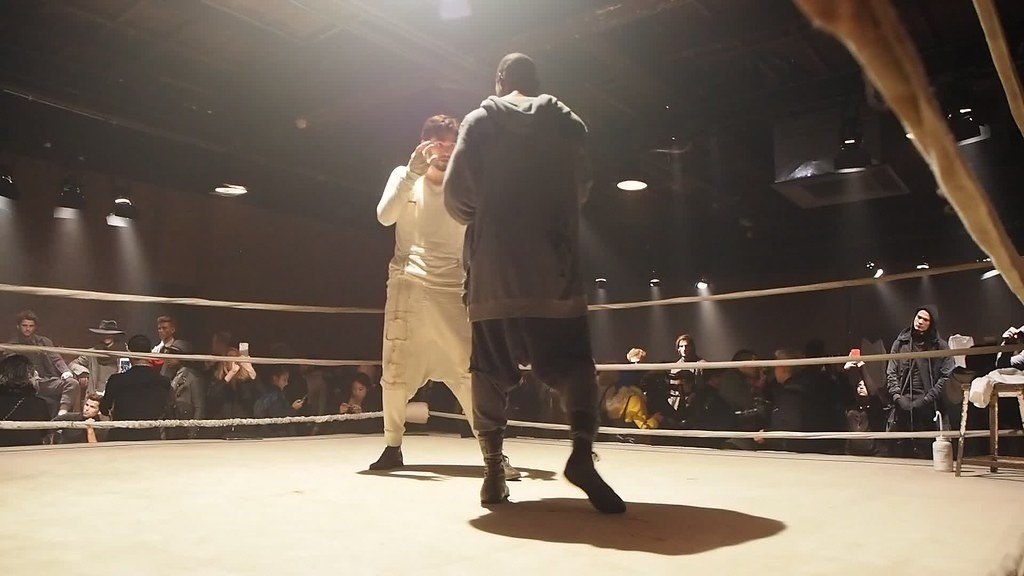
[443,53,625,515]
[367,115,521,480]
[0,305,1024,459]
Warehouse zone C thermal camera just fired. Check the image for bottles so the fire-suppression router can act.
[932,436,953,472]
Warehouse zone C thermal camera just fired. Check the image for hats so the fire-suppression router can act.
[88,320,126,334]
[165,340,194,364]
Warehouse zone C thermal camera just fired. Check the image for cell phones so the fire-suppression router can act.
[850,349,860,357]
[119,357,130,373]
[303,394,308,402]
[239,343,249,357]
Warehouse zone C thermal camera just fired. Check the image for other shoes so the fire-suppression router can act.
[502,455,521,479]
[480,474,510,503]
[564,458,626,514]
[369,452,404,470]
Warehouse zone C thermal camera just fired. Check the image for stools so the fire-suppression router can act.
[956,382,1024,478]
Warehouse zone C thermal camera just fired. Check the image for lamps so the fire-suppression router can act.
[866,258,883,279]
[107,184,137,228]
[834,118,870,176]
[946,98,990,144]
[648,267,663,288]
[0,166,20,201]
[52,162,88,218]
[975,248,1001,281]
[594,270,608,287]
[215,168,250,198]
[614,161,649,194]
[916,254,929,269]
[693,269,708,290]
